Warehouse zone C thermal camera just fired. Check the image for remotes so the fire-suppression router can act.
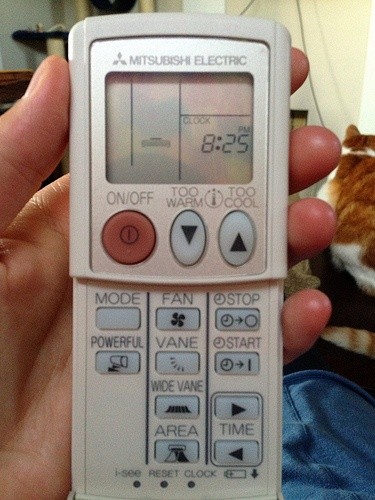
[69,12,286,500]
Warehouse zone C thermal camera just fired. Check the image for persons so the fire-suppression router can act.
[0,45,342,500]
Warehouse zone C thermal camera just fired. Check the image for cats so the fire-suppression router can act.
[316,125,375,302]
